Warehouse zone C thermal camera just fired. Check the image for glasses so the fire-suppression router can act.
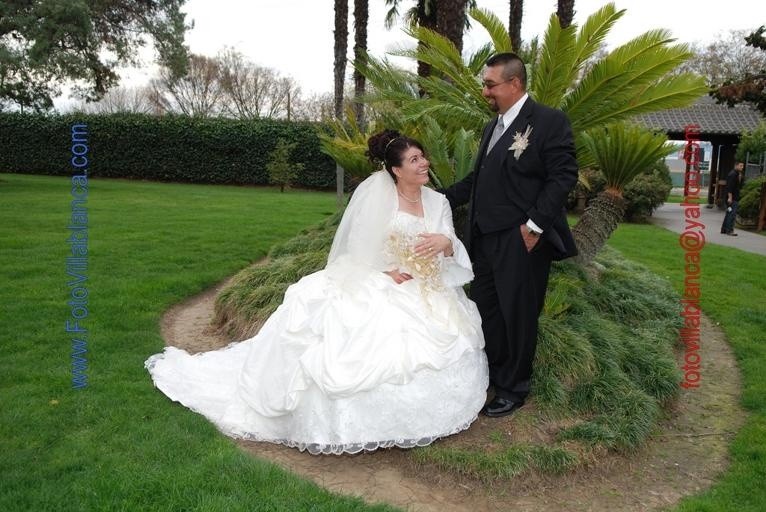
[481,80,512,88]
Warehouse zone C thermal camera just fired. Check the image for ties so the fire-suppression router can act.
[487,115,504,157]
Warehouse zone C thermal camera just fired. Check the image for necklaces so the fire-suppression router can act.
[398,188,421,203]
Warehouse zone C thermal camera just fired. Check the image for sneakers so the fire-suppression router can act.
[721,230,739,236]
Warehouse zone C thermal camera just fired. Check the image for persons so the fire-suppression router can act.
[720,161,746,236]
[144,129,491,458]
[433,50,580,418]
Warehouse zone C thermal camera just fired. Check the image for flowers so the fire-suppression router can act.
[507,124,533,161]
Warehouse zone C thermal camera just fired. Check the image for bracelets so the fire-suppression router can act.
[525,224,541,238]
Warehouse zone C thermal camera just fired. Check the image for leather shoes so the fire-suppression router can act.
[482,396,525,418]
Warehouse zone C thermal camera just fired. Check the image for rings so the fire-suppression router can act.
[428,248,432,252]
